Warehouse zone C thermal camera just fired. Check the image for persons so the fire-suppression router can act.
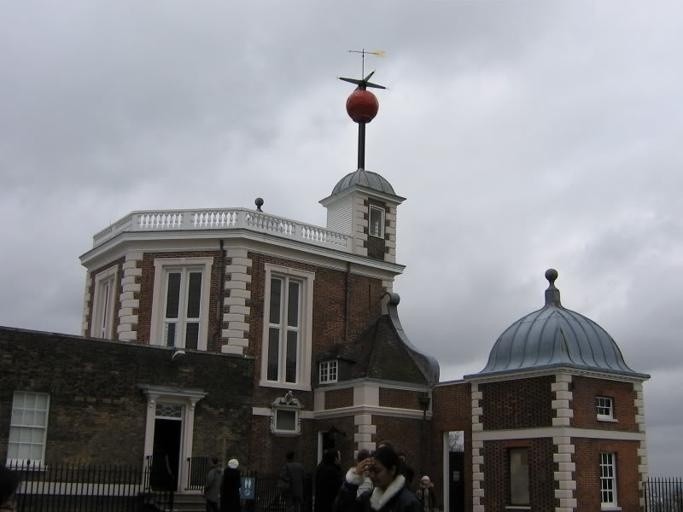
[354,448,373,500]
[203,455,224,512]
[313,448,346,511]
[218,458,243,512]
[415,475,441,511]
[398,453,408,465]
[277,449,311,512]
[376,439,394,452]
[333,447,421,511]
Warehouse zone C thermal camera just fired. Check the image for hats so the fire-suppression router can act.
[421,476,431,482]
[227,459,240,469]
